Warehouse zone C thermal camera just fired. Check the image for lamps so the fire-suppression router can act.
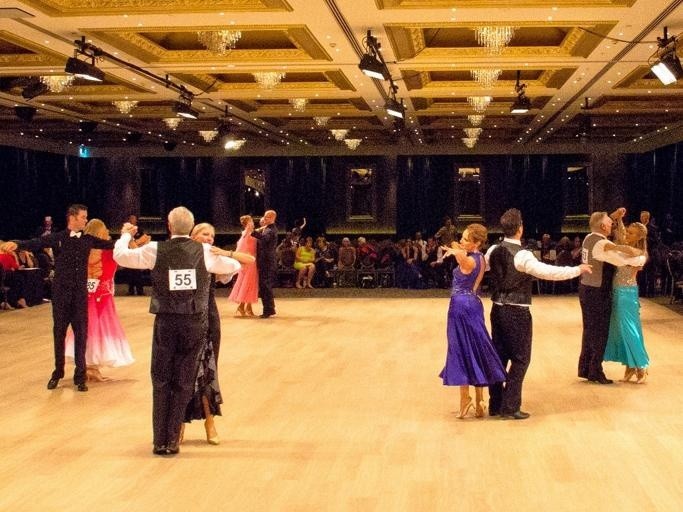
[650,27,683,86]
[461,26,515,149]
[357,54,388,81]
[580,98,592,135]
[511,77,527,113]
[383,96,404,121]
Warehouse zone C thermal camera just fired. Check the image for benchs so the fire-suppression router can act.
[334,269,396,288]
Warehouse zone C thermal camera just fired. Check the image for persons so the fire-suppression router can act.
[441,223,509,418]
[120,221,256,445]
[112,205,241,455]
[522,234,581,296]
[234,215,259,318]
[605,207,649,384]
[1,205,147,391]
[641,210,675,294]
[578,212,647,384]
[483,208,593,418]
[84,219,151,381]
[2,216,59,308]
[127,214,145,295]
[248,210,278,319]
[277,218,457,290]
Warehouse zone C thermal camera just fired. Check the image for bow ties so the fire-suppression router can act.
[69,230,82,238]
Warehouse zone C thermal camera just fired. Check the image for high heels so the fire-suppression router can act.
[455,395,476,419]
[473,400,486,418]
[619,368,639,382]
[236,306,257,318]
[204,420,220,445]
[86,368,109,382]
[0,298,52,313]
[633,367,648,384]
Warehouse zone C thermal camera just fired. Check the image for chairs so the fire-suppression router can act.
[530,250,573,295]
[667,253,683,304]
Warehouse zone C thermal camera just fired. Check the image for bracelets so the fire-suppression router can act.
[229,250,233,257]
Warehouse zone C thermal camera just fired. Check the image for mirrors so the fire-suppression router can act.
[562,162,592,221]
[453,162,486,222]
[345,163,378,224]
[238,163,271,224]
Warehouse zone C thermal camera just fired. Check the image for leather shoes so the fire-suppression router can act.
[153,443,167,454]
[588,376,613,384]
[165,441,178,454]
[489,410,529,419]
[78,383,88,391]
[260,312,276,318]
[48,377,60,389]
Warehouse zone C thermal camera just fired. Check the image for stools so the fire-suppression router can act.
[278,271,297,288]
